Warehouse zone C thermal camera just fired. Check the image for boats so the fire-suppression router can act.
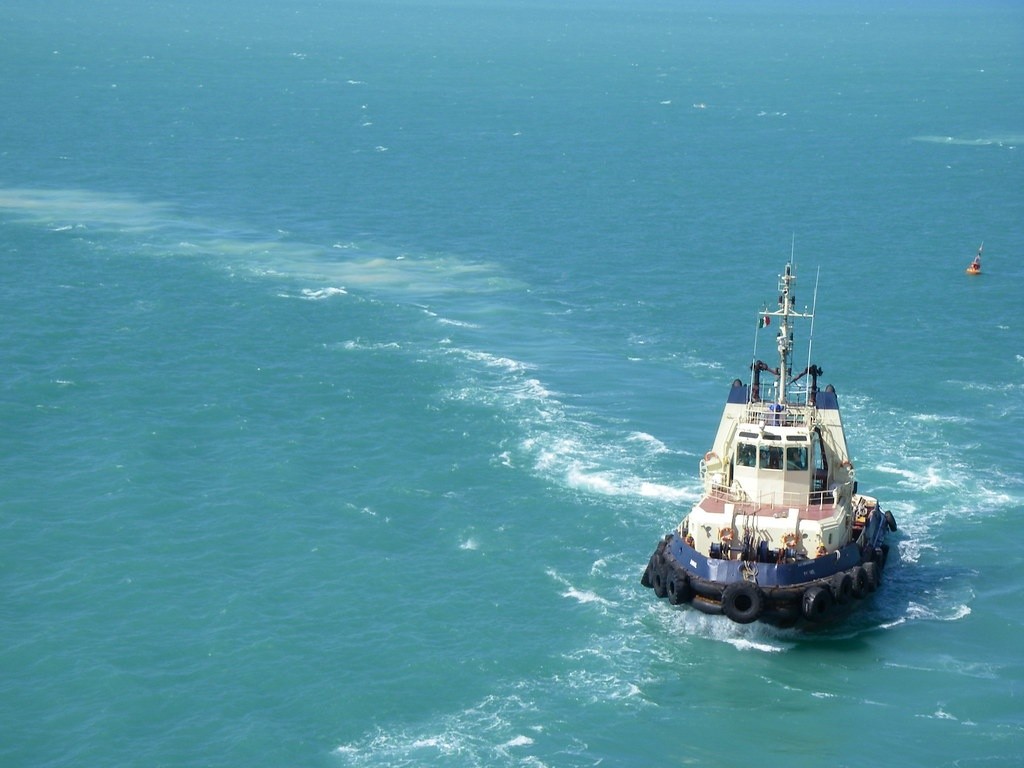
[966,241,985,273]
[639,226,901,643]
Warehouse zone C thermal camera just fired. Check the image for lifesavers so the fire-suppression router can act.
[648,534,689,605]
[885,510,898,533]
[720,527,735,542]
[716,544,884,628]
[784,533,798,547]
